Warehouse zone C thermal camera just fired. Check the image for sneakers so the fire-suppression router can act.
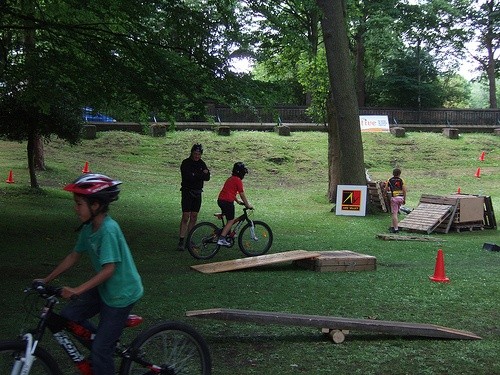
[217,237,230,246]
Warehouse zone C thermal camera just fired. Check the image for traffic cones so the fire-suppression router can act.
[456,187,461,194]
[474,167,481,179]
[427,249,450,283]
[480,152,485,160]
[6,169,15,183]
[82,160,90,173]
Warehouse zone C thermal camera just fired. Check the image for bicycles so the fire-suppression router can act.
[185,202,277,262]
[0,279,214,375]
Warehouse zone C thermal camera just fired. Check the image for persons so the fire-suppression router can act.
[177,143,210,251]
[384,168,407,233]
[32,173,144,375]
[217,162,253,246]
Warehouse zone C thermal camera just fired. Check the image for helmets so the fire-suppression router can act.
[232,162,248,179]
[191,143,203,153]
[63,174,123,202]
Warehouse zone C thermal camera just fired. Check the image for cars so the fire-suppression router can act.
[80,106,117,123]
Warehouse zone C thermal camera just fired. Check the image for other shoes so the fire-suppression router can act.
[187,239,195,247]
[177,241,185,251]
[390,227,399,233]
